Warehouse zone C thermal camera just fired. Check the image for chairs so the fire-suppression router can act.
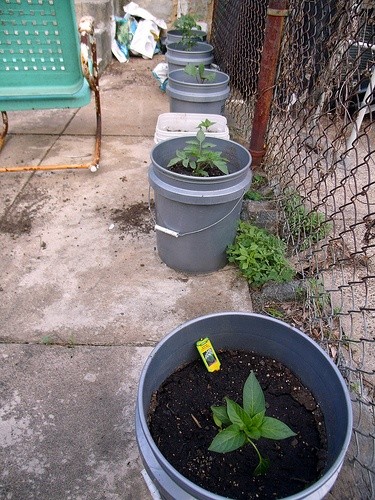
[0,0,102,172]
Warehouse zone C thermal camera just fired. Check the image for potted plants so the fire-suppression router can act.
[134,13,353,500]
